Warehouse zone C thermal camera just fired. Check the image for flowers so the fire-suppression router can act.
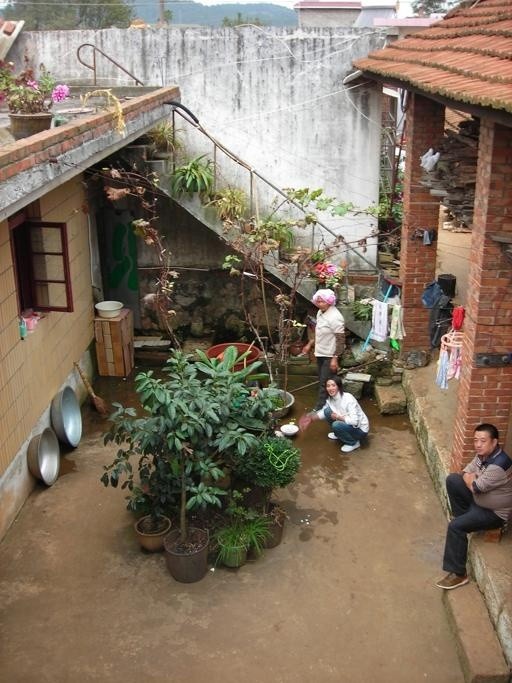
[0,60,71,114]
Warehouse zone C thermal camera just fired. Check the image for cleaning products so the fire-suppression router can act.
[19,315,26,337]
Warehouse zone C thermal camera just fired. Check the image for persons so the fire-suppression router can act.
[299,376,370,453]
[435,424,512,590]
[302,288,345,417]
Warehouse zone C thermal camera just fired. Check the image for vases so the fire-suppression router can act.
[9,115,53,140]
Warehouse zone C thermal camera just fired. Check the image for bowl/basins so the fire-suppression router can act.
[28,385,83,487]
[281,425,299,436]
[95,300,123,318]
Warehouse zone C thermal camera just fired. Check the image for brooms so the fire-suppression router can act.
[75,361,110,417]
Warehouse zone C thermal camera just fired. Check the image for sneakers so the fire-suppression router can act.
[435,573,470,590]
[341,440,360,452]
[328,432,338,439]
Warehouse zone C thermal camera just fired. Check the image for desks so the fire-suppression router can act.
[93,309,135,377]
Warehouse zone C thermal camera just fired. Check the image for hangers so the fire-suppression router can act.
[439,328,463,350]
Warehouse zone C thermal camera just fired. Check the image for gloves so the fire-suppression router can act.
[299,415,315,432]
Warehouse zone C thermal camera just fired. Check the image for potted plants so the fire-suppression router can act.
[100,344,303,582]
[144,117,188,160]
[216,187,249,220]
[169,152,220,198]
